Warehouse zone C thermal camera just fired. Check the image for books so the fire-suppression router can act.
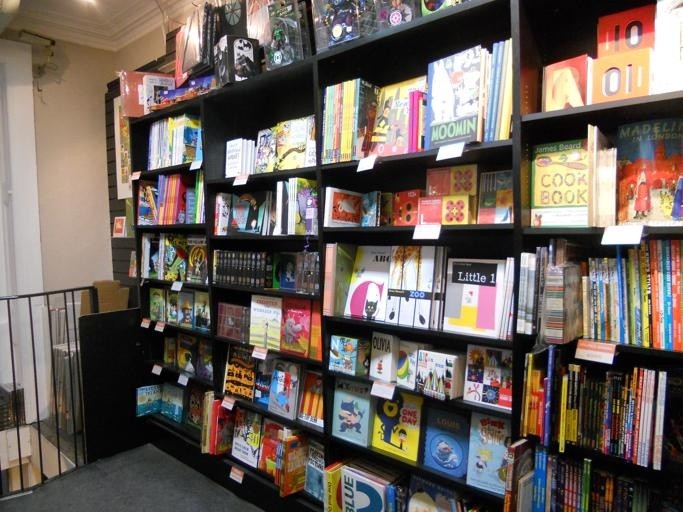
[521,113,683,228]
[225,114,316,177]
[2,382,25,429]
[213,250,320,295]
[216,294,322,360]
[223,342,325,427]
[512,345,682,460]
[214,177,318,236]
[323,452,502,510]
[164,328,213,382]
[516,237,683,353]
[149,287,211,331]
[324,164,515,226]
[328,331,512,411]
[140,232,209,286]
[332,375,512,496]
[322,242,514,340]
[541,1,682,112]
[320,37,514,165]
[133,72,175,117]
[143,75,175,116]
[136,382,325,503]
[504,440,681,510]
[148,113,202,170]
[137,170,206,225]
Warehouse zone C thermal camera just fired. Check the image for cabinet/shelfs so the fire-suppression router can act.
[515,0,683,512]
[128,101,216,447]
[204,59,326,512]
[315,0,514,512]
